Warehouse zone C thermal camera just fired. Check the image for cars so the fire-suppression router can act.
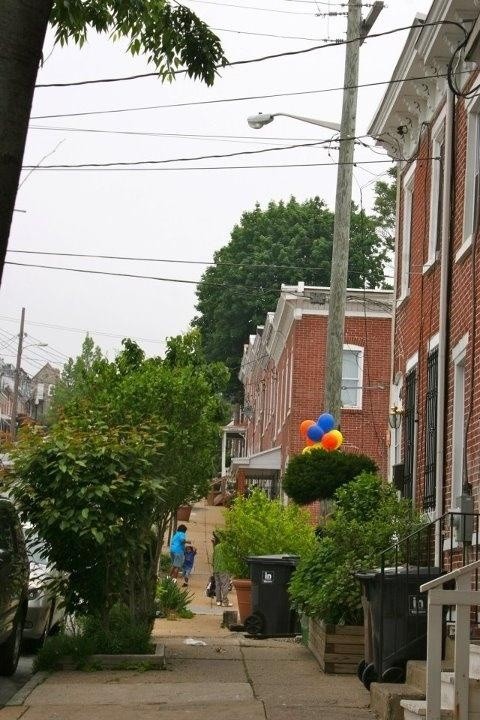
[0,451,72,677]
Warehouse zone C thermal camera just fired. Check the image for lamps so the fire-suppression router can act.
[388,403,404,429]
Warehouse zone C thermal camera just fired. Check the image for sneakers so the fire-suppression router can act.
[182,577,188,587]
[167,575,178,584]
[217,601,233,606]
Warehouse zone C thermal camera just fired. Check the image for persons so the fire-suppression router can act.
[211,530,233,607]
[169,525,197,587]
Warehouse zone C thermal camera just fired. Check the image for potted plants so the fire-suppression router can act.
[287,470,428,674]
[214,486,318,625]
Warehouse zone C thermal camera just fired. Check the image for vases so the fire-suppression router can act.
[177,505,192,521]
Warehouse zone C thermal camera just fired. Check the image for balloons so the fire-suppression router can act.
[301,413,343,455]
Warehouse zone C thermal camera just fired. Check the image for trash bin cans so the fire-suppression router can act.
[244,554,300,638]
[355,565,456,691]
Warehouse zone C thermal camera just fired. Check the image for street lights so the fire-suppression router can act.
[9,343,50,438]
[244,107,356,529]
